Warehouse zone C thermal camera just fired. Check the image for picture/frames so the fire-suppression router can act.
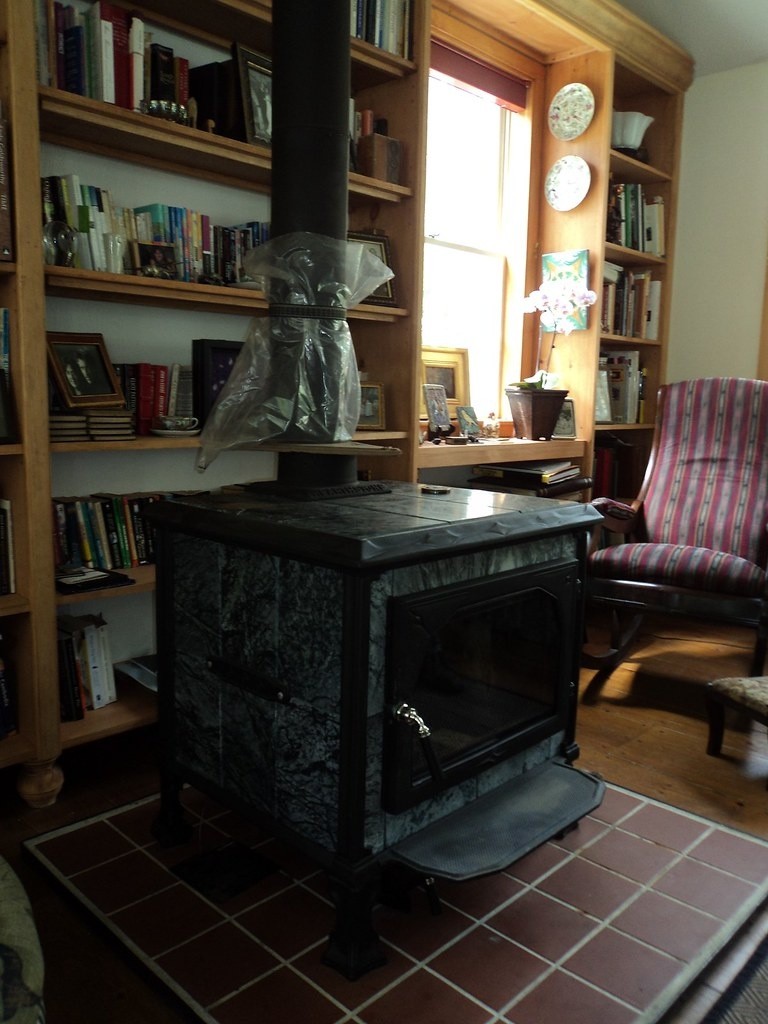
[419,346,470,419]
[552,399,577,439]
[128,240,183,281]
[356,381,385,429]
[424,385,451,432]
[347,231,399,307]
[231,40,271,149]
[46,332,126,408]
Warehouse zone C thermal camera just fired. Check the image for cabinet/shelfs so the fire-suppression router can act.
[0,0,696,810]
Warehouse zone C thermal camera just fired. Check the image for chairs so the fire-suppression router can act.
[582,377,768,707]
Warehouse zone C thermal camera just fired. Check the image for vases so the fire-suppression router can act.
[505,389,569,440]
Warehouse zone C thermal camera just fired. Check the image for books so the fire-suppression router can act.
[0,123,23,595]
[31,0,242,137]
[601,261,661,340]
[600,350,646,423]
[49,363,194,443]
[468,461,593,501]
[53,481,252,596]
[606,184,665,257]
[39,174,270,288]
[349,0,412,172]
[0,613,158,741]
[593,446,618,499]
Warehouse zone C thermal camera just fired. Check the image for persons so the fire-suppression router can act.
[429,390,443,422]
[361,391,373,417]
[256,83,272,135]
[554,413,570,433]
[150,247,175,269]
[62,351,108,395]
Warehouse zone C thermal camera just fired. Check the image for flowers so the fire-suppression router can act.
[519,279,597,387]
